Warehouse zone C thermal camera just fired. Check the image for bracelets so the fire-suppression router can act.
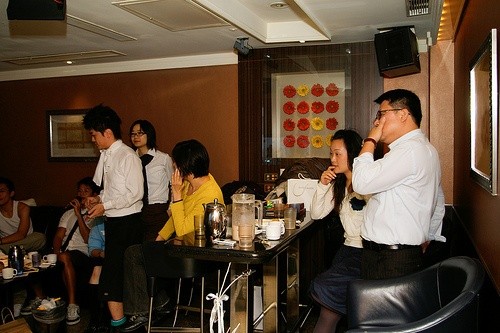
[364,138,377,146]
[173,199,183,203]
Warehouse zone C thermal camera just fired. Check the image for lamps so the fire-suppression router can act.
[232,37,252,54]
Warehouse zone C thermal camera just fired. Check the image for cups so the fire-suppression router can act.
[284,208,297,229]
[28,252,40,268]
[238,223,253,247]
[194,214,206,235]
[43,254,57,264]
[274,203,285,217]
[266,221,286,240]
[2,268,17,279]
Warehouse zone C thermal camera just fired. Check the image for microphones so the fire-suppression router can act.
[65,196,82,208]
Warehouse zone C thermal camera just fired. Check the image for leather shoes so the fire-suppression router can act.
[362,239,422,250]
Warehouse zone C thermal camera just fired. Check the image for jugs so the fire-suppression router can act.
[202,198,227,240]
[230,193,263,241]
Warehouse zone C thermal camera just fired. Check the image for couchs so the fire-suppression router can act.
[342,256,489,333]
[29,205,67,250]
[418,213,456,265]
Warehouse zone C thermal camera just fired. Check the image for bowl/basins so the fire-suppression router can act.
[32,299,66,324]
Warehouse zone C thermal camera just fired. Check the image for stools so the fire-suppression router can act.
[142,239,204,333]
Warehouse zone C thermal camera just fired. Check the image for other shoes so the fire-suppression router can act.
[118,303,175,333]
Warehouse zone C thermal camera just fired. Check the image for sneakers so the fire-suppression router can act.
[66,304,80,325]
[20,296,43,315]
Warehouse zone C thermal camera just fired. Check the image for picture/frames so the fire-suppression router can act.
[44,108,101,163]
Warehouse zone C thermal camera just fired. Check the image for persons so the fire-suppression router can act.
[81,105,144,333]
[20,177,105,326]
[120,119,226,330]
[0,182,47,320]
[352,89,447,333]
[309,128,385,333]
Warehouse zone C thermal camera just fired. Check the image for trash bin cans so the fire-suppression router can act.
[30,298,69,333]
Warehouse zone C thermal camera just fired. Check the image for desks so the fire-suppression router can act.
[162,213,314,333]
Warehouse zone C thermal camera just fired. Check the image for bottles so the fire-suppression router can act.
[8,244,24,274]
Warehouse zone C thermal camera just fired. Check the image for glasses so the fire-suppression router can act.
[129,131,145,137]
[376,109,411,120]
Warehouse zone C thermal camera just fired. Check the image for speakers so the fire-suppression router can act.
[374,28,421,78]
[6,0,66,20]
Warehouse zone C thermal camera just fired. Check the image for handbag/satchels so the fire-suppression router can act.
[285,173,320,212]
